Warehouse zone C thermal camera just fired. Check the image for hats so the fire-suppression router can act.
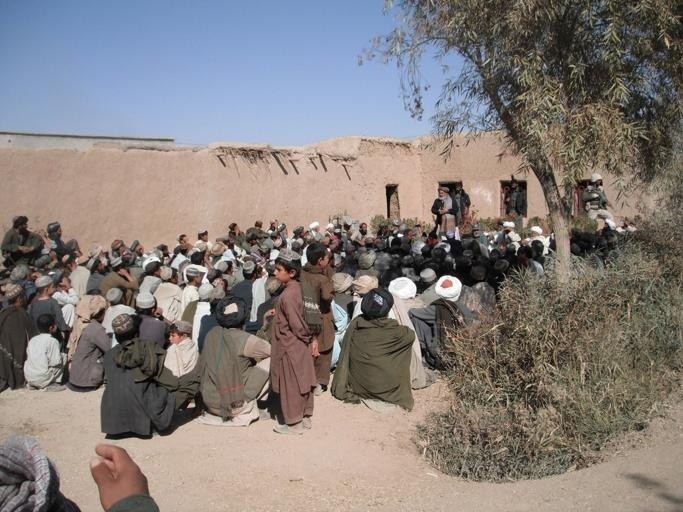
[5,217,543,333]
[591,173,603,182]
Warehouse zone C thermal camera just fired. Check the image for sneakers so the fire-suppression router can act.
[273,421,304,434]
[303,417,311,429]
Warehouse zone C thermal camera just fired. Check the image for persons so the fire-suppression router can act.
[0,214,639,436]
[581,173,615,232]
[503,179,524,219]
[0,436,160,511]
[431,187,458,233]
[453,185,471,227]
